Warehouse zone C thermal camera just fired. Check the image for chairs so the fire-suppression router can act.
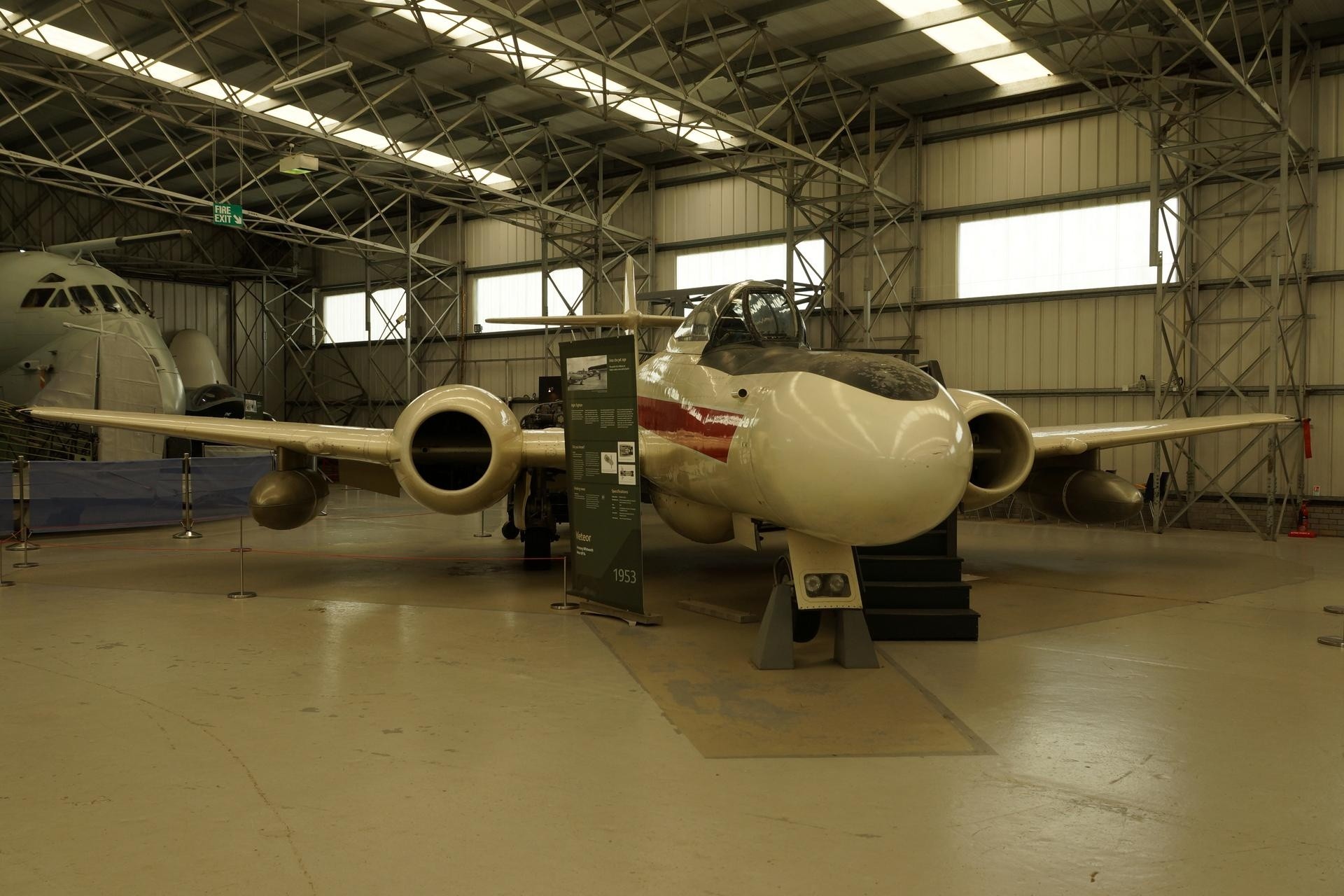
[706,303,749,346]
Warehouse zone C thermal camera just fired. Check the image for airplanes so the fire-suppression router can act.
[0,225,268,517]
[8,276,1314,674]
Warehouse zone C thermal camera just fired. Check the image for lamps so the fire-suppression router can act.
[272,0,353,92]
